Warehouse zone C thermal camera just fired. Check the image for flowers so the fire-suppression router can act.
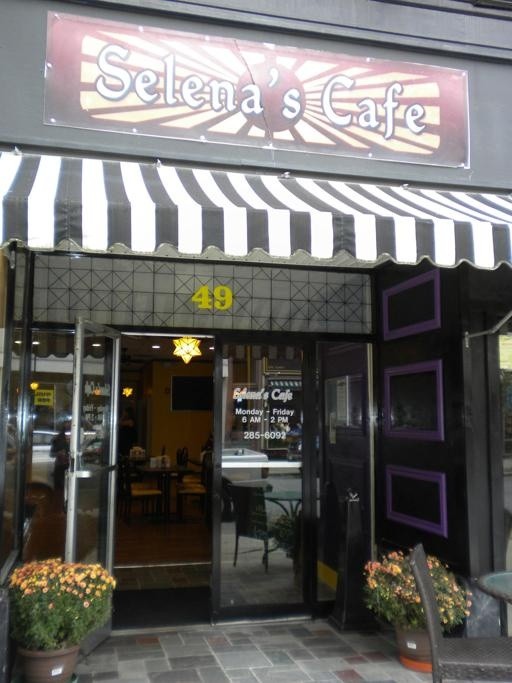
[358,548,474,633]
[5,553,116,653]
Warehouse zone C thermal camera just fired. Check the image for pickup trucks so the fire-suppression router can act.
[219,434,321,523]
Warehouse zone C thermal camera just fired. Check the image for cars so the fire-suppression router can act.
[23,426,98,520]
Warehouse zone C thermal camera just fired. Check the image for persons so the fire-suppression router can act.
[118,406,138,454]
[51,427,69,489]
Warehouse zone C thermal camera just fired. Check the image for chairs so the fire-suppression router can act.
[227,482,285,573]
[118,446,213,532]
[406,542,512,682]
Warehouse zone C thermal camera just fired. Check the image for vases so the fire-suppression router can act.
[16,645,81,682]
[391,619,444,672]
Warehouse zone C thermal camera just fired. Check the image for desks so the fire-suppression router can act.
[262,490,321,563]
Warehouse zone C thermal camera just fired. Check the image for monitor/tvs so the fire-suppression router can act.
[172,376,213,410]
